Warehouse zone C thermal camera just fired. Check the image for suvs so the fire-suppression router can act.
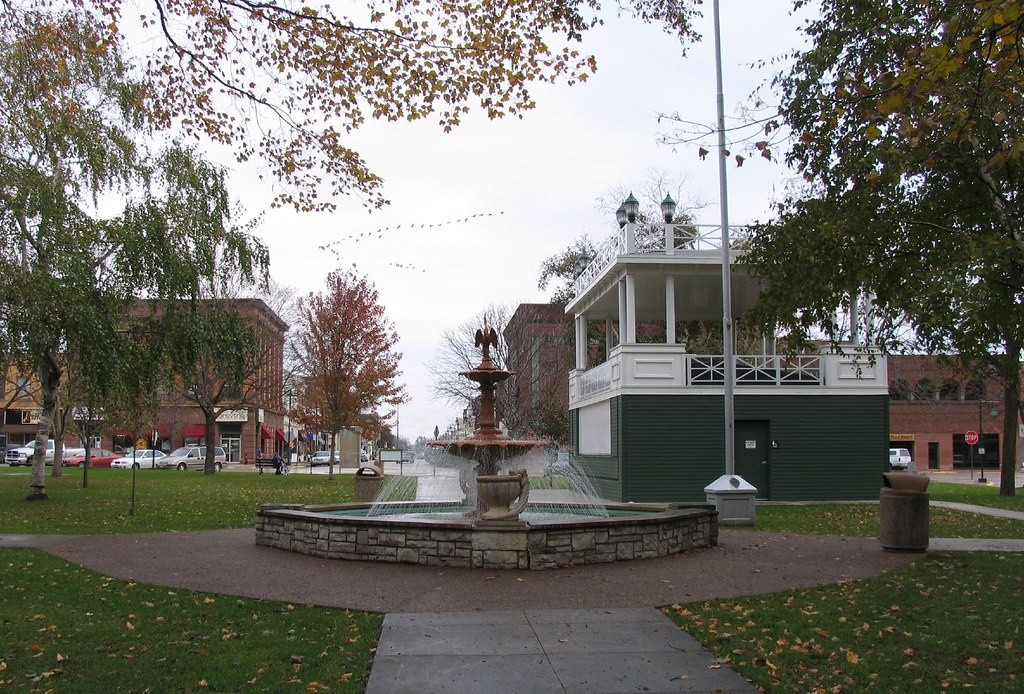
[155,446,227,473]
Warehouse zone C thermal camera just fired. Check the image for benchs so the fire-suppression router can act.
[255,458,290,475]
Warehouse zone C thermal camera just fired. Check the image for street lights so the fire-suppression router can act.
[660,191,677,342]
[623,191,639,344]
[976,365,987,483]
[616,200,628,344]
[287,388,294,466]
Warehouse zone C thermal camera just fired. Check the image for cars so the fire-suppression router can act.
[309,448,370,467]
[66,448,123,469]
[110,449,167,470]
[396,451,415,463]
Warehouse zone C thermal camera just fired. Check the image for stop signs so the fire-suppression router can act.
[965,431,978,445]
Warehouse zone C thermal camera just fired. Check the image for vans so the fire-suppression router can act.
[889,448,912,471]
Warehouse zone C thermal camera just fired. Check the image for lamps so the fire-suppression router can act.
[990,407,999,417]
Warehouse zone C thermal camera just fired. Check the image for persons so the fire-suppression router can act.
[256,447,263,459]
[306,448,310,462]
[273,453,284,472]
[244,454,248,464]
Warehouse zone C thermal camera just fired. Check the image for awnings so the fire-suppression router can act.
[185,424,206,437]
[261,427,274,439]
[299,430,307,442]
[276,429,285,440]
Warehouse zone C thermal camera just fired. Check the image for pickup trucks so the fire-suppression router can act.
[4,439,86,468]
